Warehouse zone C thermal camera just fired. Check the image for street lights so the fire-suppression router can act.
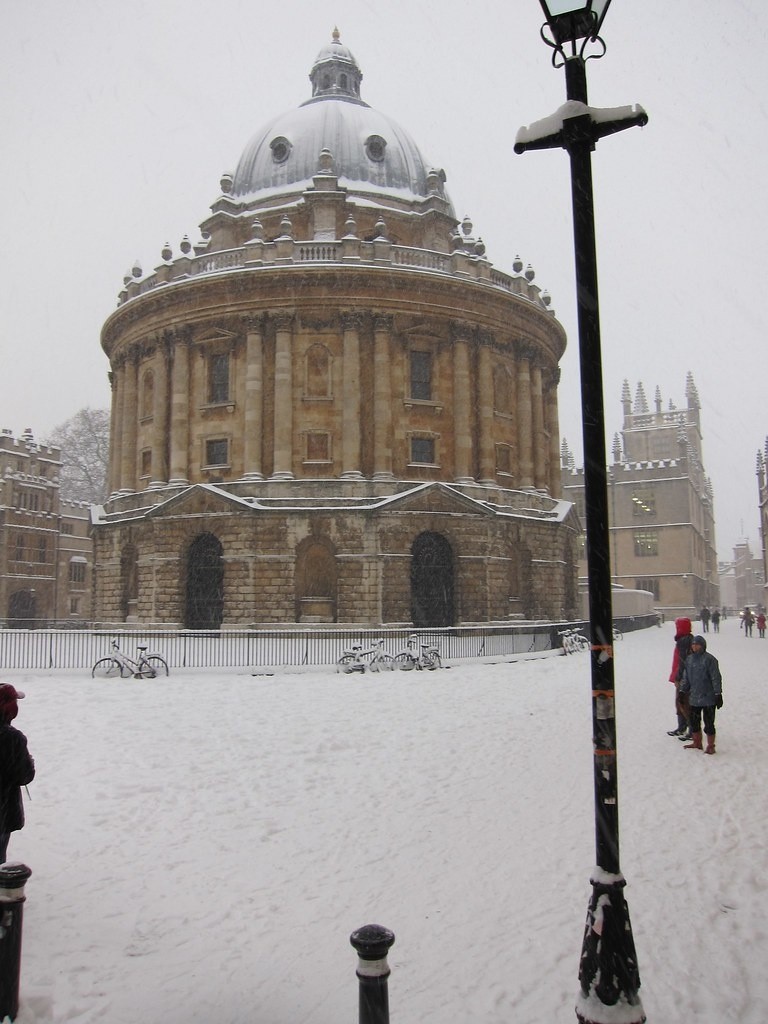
[510,0,653,1024]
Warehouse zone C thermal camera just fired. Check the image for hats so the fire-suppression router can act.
[0,682,25,704]
[691,641,699,645]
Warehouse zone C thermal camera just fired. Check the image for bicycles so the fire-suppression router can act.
[391,635,442,672]
[612,623,623,642]
[91,637,169,680]
[557,628,590,656]
[336,638,398,676]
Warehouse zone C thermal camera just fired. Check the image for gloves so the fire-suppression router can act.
[714,694,723,710]
[678,691,686,705]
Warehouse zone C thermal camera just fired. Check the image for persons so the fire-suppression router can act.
[668,617,693,736]
[0,683,35,864]
[679,635,723,754]
[712,610,720,632]
[756,613,766,638]
[700,605,711,633]
[722,606,726,619]
[740,607,755,637]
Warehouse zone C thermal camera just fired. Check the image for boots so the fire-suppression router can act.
[667,713,688,736]
[678,727,694,741]
[704,734,716,754]
[683,731,703,749]
